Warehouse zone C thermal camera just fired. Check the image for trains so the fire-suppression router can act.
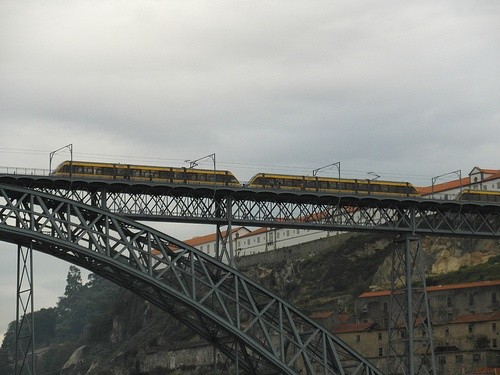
[250,174,420,197]
[51,160,241,186]
[455,188,500,203]
